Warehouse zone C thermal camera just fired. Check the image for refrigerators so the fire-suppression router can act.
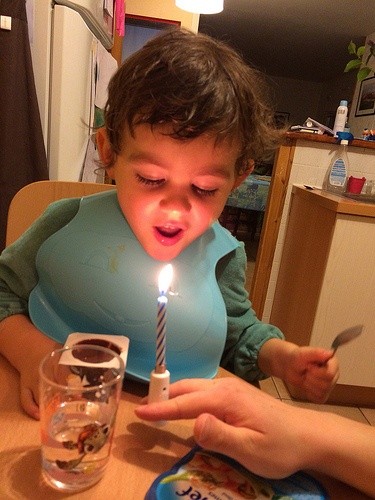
[24,0,115,183]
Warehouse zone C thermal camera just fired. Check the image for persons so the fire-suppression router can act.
[135,377,375,498]
[0,28,341,421]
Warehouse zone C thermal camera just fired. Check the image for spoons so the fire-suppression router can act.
[316,324,364,367]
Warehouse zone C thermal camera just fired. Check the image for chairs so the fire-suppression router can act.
[5,179,118,251]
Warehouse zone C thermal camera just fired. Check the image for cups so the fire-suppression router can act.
[58,332,130,378]
[38,344,126,493]
[346,176,366,194]
[363,180,375,197]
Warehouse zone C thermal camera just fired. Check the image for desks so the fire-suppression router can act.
[0,352,375,500]
[225,174,272,242]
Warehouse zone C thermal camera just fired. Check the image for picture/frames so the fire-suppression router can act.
[354,76,375,118]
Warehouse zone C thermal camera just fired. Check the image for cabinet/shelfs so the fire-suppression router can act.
[269,183,375,410]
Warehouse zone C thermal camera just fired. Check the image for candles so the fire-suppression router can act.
[154,263,176,371]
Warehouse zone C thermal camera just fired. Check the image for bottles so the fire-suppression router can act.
[333,100,349,137]
